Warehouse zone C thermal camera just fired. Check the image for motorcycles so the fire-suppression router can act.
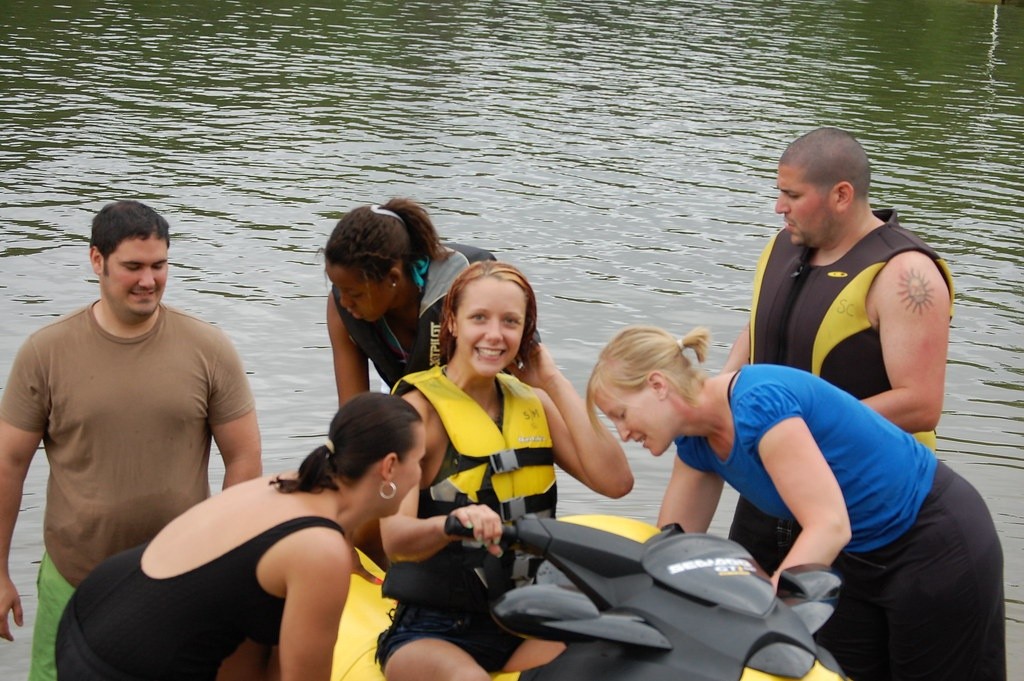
[309,515,854,681]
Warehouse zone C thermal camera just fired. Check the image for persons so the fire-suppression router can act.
[725,128,955,574]
[380,260,633,680]
[326,198,499,409]
[0,202,262,681]
[54,393,425,680]
[586,326,1007,681]
